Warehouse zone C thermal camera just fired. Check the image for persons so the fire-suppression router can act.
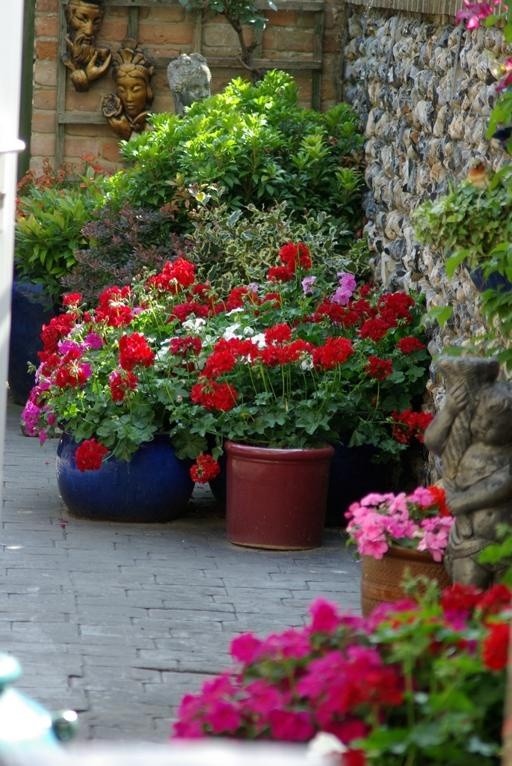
[168,53,212,115]
[424,379,511,589]
[102,48,154,140]
[63,0,111,91]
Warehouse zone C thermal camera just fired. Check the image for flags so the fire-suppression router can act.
[56,435,196,523]
[360,547,451,619]
[223,439,335,549]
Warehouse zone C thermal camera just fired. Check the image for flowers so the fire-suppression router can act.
[151,242,429,483]
[171,583,512,764]
[345,483,456,564]
[22,282,174,470]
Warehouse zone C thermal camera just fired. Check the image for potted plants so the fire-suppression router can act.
[420,162,512,372]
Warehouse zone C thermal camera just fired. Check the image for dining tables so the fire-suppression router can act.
[420,162,512,372]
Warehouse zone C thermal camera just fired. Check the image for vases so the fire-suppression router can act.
[345,483,456,564]
[151,242,429,483]
[360,547,451,619]
[56,435,196,523]
[22,282,174,470]
[223,439,335,549]
